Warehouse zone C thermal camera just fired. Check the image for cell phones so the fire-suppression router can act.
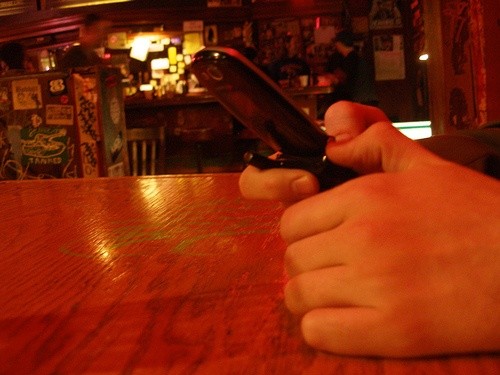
[191,46,364,193]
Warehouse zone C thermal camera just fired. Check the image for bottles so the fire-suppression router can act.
[309,67,315,88]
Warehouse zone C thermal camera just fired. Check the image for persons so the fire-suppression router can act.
[317,29,373,120]
[237,99,500,359]
[0,41,25,74]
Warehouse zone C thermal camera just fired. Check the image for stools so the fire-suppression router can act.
[181,127,214,172]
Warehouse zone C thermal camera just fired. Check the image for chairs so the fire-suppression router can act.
[125,127,164,177]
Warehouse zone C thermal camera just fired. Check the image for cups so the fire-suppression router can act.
[299,76,309,87]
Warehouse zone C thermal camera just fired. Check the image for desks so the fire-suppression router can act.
[1,171,499,372]
[124,88,333,142]
